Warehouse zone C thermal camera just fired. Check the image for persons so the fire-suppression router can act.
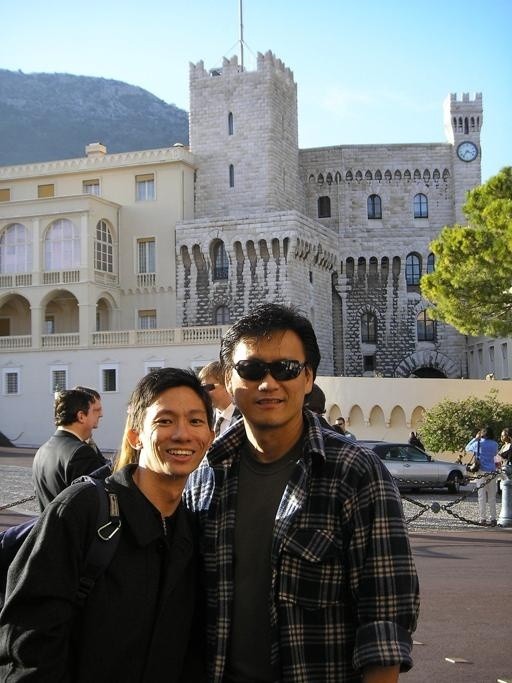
[31,386,105,514]
[498,426,512,481]
[181,302,422,682]
[465,428,498,527]
[198,360,242,443]
[334,417,356,442]
[0,365,218,683]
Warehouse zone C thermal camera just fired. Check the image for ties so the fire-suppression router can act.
[215,417,223,437]
[89,439,95,454]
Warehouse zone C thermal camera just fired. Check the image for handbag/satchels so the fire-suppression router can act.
[1,475,121,683]
[466,461,480,474]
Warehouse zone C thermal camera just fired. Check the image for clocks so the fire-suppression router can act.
[457,142,478,162]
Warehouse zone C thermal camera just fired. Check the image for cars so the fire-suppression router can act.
[355,440,467,491]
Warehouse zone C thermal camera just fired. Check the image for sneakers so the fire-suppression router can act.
[479,520,503,528]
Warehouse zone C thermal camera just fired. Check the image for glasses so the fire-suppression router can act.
[335,423,344,425]
[231,361,305,381]
[201,384,221,391]
